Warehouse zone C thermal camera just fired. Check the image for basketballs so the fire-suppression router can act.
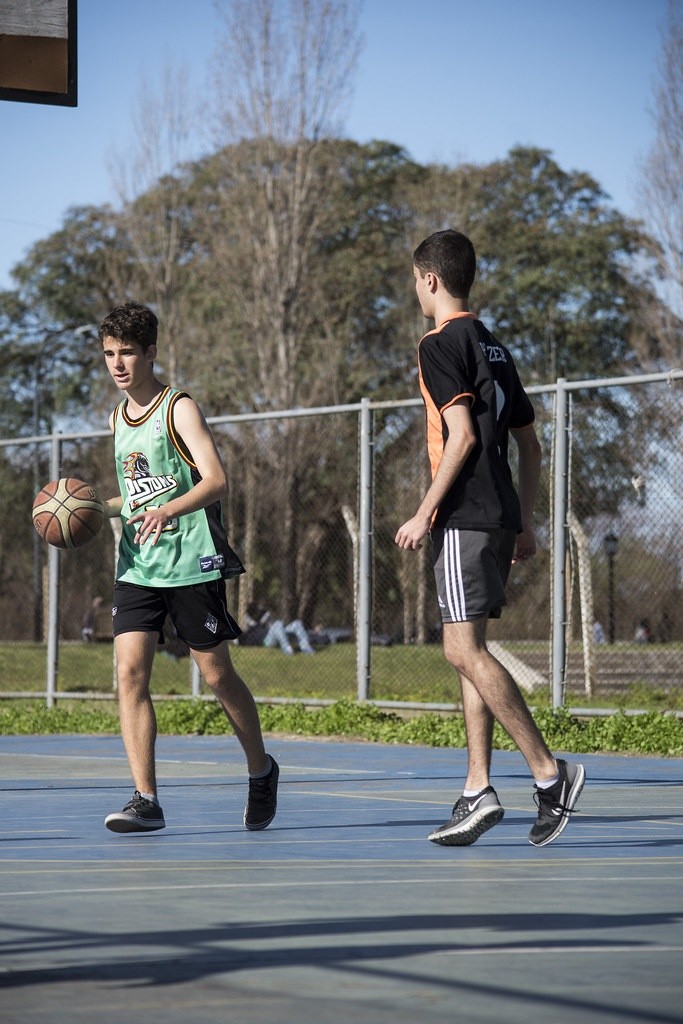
[32,477,105,550]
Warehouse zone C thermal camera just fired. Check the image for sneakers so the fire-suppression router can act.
[528,758,586,846]
[104,791,166,833]
[244,753,279,831]
[427,786,504,846]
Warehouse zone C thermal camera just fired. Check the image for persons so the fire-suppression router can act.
[634,626,651,645]
[97,300,280,833]
[393,230,587,848]
[585,615,610,648]
[230,598,317,658]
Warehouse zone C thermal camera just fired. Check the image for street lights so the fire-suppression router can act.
[603,530,620,648]
